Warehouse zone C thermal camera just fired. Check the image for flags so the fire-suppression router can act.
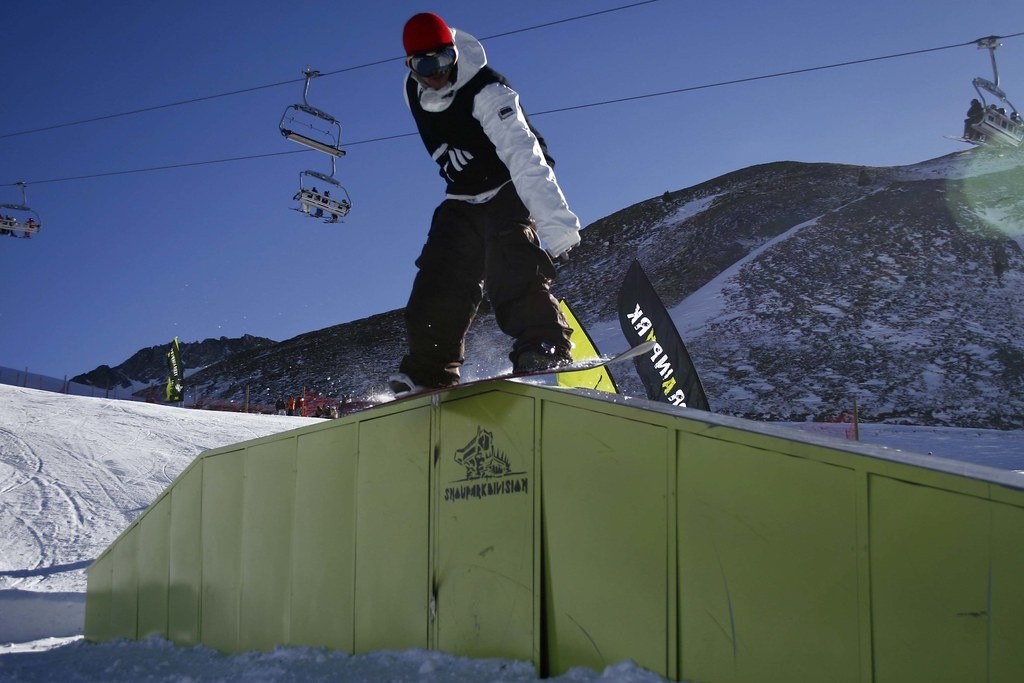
[552,300,620,394]
[164,335,186,403]
[616,257,712,413]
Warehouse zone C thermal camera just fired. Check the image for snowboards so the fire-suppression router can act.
[337,338,658,417]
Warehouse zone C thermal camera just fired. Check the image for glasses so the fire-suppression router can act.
[407,45,458,77]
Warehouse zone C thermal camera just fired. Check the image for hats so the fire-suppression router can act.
[403,13,452,56]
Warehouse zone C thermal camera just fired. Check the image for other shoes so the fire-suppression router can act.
[509,338,573,370]
[388,355,460,393]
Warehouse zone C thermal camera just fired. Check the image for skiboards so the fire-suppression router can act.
[942,135,1006,152]
[287,205,347,226]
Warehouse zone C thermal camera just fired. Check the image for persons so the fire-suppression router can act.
[275,395,338,419]
[962,99,1024,142]
[388,11,582,402]
[0,214,38,239]
[299,187,349,223]
